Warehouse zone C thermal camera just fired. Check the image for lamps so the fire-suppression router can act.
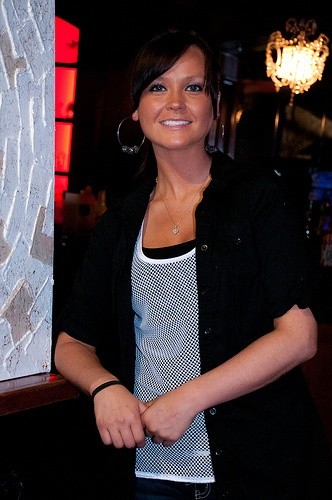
[265,17,330,110]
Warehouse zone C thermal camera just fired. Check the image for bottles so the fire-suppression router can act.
[302,190,332,268]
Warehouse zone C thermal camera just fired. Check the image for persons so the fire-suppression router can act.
[52,30,320,500]
[276,77,332,169]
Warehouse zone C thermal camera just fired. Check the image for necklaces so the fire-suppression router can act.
[156,175,209,234]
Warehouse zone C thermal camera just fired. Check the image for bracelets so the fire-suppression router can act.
[320,116,326,137]
[91,379,123,396]
